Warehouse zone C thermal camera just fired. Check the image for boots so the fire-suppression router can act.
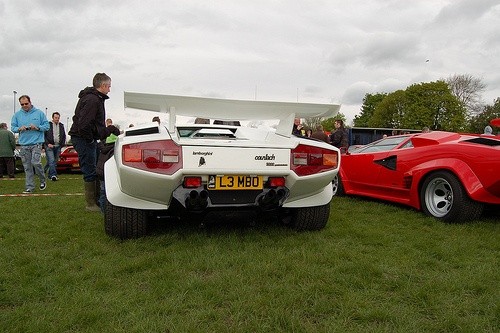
[84,181,101,211]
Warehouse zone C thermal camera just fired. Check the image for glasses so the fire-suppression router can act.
[20,102,29,106]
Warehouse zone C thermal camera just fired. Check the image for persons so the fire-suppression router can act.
[292,119,350,150]
[379,127,430,138]
[68,73,111,212]
[42,112,65,181]
[483,126,495,136]
[0,123,15,178]
[10,95,50,193]
[100,118,120,148]
[43,162,51,177]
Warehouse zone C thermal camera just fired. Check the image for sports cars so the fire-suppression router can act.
[53,146,83,172]
[100,90,345,239]
[333,131,500,224]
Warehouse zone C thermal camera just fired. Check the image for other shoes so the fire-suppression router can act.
[22,188,32,194]
[1,176,7,181]
[9,175,16,180]
[39,182,47,191]
[51,176,58,181]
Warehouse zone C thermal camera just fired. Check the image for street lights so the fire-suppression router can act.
[13,90,18,114]
